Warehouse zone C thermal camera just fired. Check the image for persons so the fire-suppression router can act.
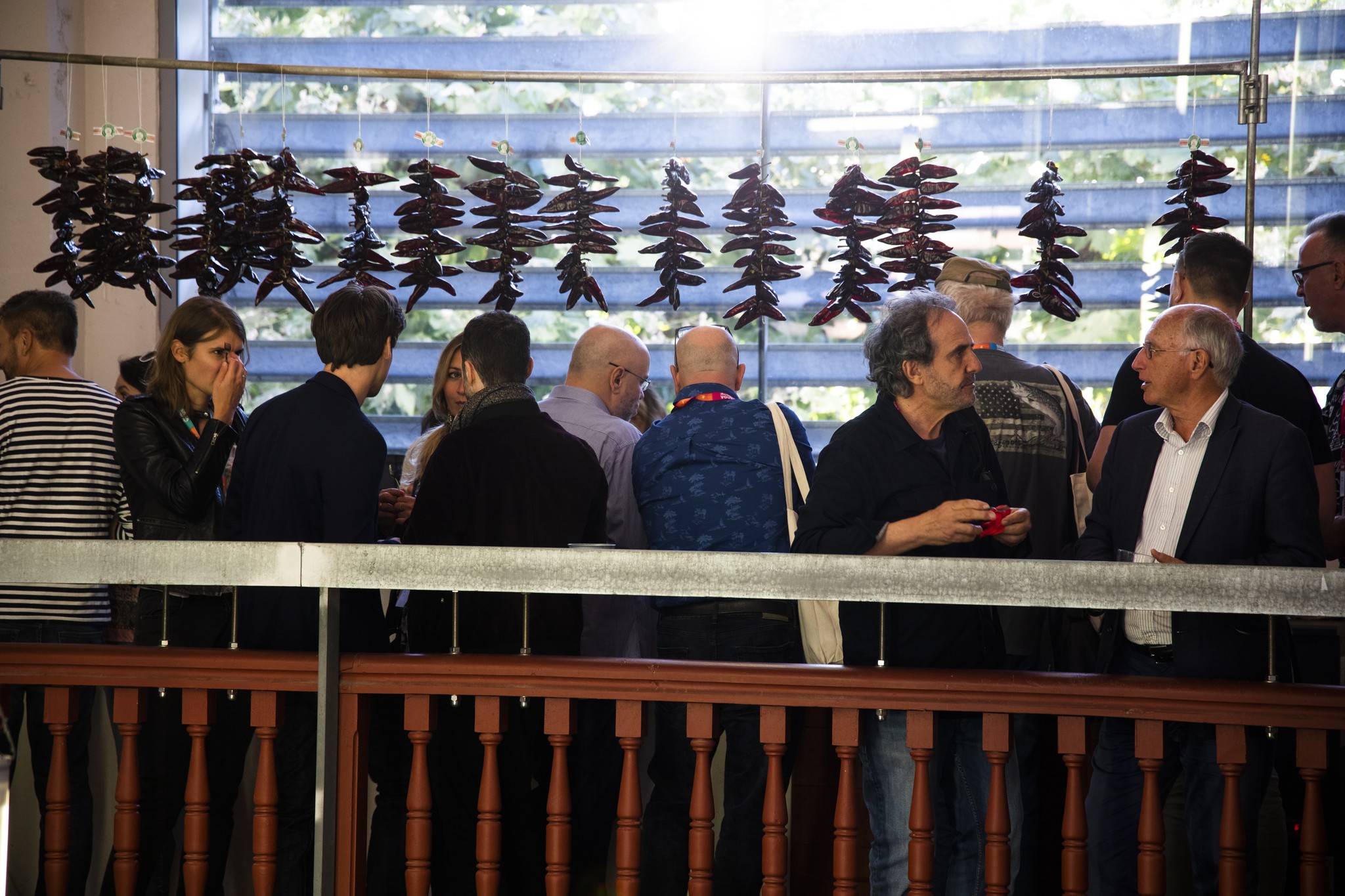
[221,286,413,896]
[1080,233,1339,895]
[930,256,1103,896]
[1076,303,1324,895]
[101,294,255,896]
[406,312,610,895]
[113,351,156,405]
[790,292,1035,895]
[1295,212,1345,896]
[534,322,648,895]
[0,289,134,896]
[396,332,471,537]
[631,325,819,896]
[627,385,668,434]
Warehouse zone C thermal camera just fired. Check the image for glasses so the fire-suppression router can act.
[674,325,739,373]
[1292,260,1334,287]
[1183,236,1193,280]
[139,349,158,363]
[609,362,651,391]
[1143,343,1214,368]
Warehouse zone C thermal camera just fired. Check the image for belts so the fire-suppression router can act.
[1125,638,1175,665]
[659,599,796,618]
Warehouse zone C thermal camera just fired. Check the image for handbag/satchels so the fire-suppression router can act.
[1040,362,1093,537]
[765,400,842,665]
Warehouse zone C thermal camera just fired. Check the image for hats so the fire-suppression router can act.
[935,256,1012,294]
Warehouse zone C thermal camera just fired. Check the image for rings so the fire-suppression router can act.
[242,375,246,379]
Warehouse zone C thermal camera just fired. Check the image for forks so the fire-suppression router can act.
[389,465,401,490]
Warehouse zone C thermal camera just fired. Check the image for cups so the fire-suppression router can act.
[1116,548,1155,563]
